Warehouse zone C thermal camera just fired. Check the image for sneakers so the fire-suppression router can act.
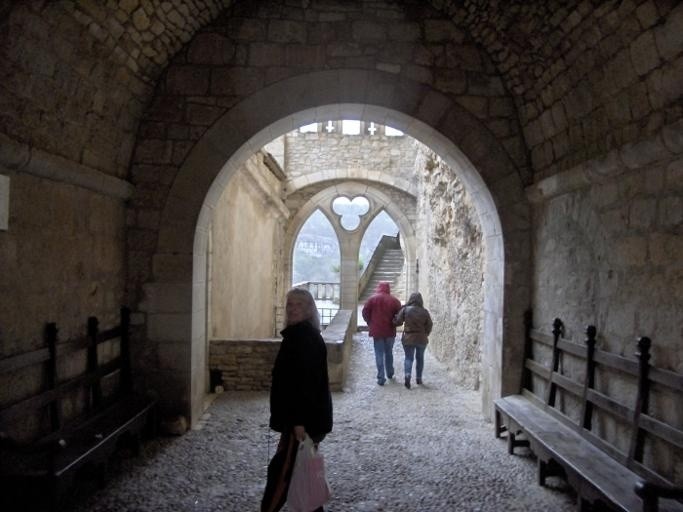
[379,376,422,388]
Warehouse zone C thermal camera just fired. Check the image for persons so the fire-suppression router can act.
[362,281,402,385]
[392,292,434,388]
[259,287,334,511]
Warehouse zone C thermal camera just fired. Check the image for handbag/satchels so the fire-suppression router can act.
[286,432,330,512]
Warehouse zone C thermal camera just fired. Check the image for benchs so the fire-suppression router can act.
[0,305,161,512]
[494,310,683,512]
